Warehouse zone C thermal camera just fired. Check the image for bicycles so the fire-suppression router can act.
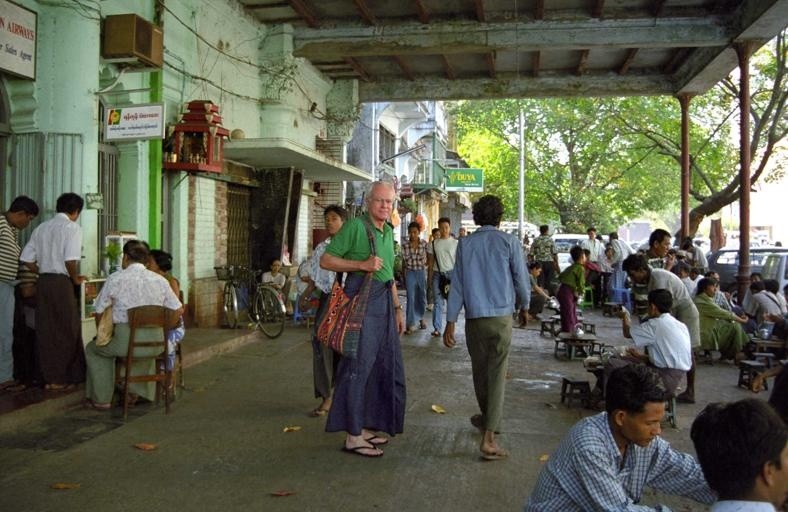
[213,265,286,339]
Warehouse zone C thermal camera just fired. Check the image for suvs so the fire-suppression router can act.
[760,253,788,293]
[707,248,788,286]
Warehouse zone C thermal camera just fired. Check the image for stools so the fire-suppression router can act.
[610,288,631,317]
[577,287,594,311]
[554,337,569,358]
[561,374,591,410]
[583,321,596,336]
[540,318,554,337]
[656,393,678,428]
[601,302,620,318]
[694,350,712,365]
[738,360,769,391]
[569,343,590,359]
[754,352,774,371]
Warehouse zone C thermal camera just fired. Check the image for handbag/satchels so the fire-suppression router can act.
[313,217,378,360]
[431,240,452,299]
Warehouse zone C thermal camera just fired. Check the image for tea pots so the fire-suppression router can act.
[572,323,584,337]
[547,297,559,307]
[755,328,768,339]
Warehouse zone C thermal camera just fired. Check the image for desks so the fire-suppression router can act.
[559,331,596,359]
[552,314,584,323]
[587,356,642,393]
[746,332,786,359]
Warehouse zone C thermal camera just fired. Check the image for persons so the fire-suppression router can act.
[0,195,39,387]
[85,240,183,410]
[623,254,700,403]
[705,271,732,312]
[678,266,696,293]
[619,289,692,397]
[321,181,407,456]
[528,263,548,320]
[691,398,787,512]
[602,248,613,267]
[743,272,763,309]
[753,361,788,420]
[425,217,466,338]
[686,269,704,281]
[581,228,602,260]
[646,229,676,272]
[431,228,441,240]
[583,249,602,308]
[404,221,428,334]
[299,205,348,417]
[455,227,465,239]
[682,241,708,271]
[694,277,749,364]
[526,364,718,512]
[261,260,286,290]
[19,192,84,392]
[148,250,185,395]
[609,231,624,265]
[762,279,787,313]
[672,250,691,276]
[557,246,585,332]
[289,257,312,318]
[443,195,531,460]
[527,225,560,289]
[745,280,782,332]
[765,284,788,341]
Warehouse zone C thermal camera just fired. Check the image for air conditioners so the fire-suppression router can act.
[102,12,166,71]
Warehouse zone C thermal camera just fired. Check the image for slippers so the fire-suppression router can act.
[0,380,27,394]
[48,382,77,392]
[85,399,112,410]
[341,441,384,457]
[344,434,390,445]
[481,446,507,461]
[310,406,330,417]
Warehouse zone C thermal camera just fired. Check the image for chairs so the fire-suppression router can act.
[167,288,184,397]
[109,305,177,422]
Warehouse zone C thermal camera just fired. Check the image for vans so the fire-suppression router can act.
[551,234,609,272]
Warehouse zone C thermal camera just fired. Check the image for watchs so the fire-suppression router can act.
[395,305,402,309]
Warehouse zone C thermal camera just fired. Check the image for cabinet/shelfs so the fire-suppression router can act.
[80,233,138,355]
